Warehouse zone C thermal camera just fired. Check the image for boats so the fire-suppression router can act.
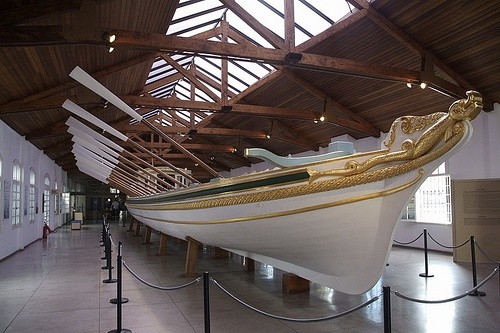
[124,61,484,297]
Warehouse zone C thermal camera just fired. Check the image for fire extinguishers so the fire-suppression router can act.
[43,222,48,240]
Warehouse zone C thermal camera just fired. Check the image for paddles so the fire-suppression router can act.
[62,98,202,185]
[72,142,161,192]
[68,66,227,178]
[71,127,168,192]
[65,115,190,189]
[67,125,177,189]
[71,148,156,196]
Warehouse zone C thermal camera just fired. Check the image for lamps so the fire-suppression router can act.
[103,31,116,53]
